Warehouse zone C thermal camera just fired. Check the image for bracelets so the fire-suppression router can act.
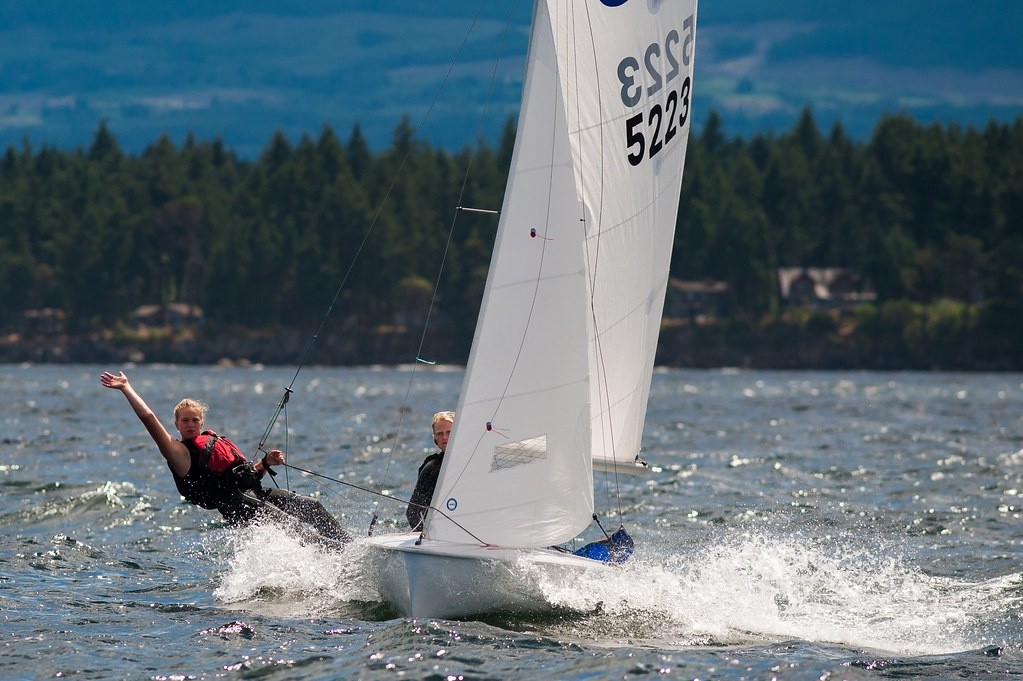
[261,456,278,477]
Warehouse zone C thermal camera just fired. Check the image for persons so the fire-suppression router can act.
[404,410,457,536]
[98,368,353,552]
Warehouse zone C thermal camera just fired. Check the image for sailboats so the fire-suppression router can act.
[350,1,699,621]
